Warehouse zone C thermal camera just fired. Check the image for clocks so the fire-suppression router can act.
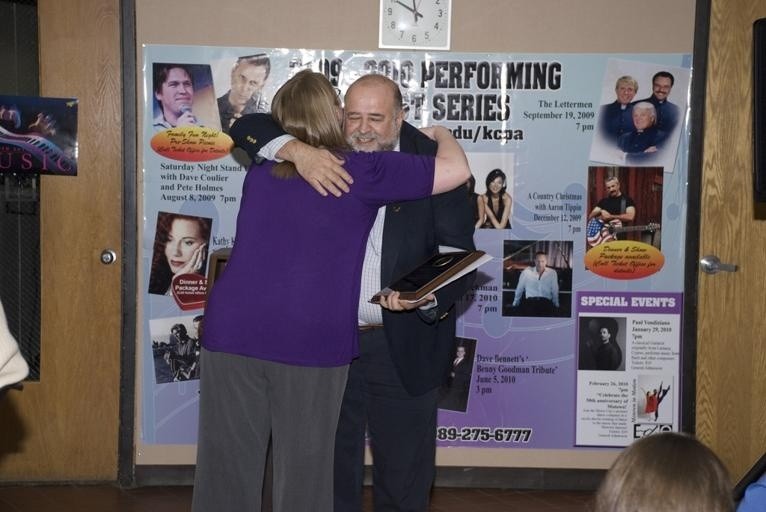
[377,1,452,52]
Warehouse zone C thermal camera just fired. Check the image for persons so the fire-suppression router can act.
[1,104,57,138]
[589,172,639,239]
[192,68,473,509]
[584,318,622,370]
[216,51,273,134]
[146,211,210,294]
[228,73,477,512]
[596,71,682,166]
[508,252,568,312]
[449,338,472,384]
[638,381,672,420]
[468,172,486,232]
[161,315,204,383]
[152,63,205,131]
[579,430,741,510]
[483,169,514,229]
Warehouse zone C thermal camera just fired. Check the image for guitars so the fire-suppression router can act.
[587,215,659,249]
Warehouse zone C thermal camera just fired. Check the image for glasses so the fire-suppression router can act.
[334,88,341,96]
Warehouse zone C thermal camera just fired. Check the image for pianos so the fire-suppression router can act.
[0,125,76,174]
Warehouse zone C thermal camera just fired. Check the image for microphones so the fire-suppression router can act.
[180,103,197,123]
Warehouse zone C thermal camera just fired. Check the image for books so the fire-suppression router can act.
[367,249,496,316]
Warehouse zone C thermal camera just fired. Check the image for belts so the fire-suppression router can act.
[357,324,383,331]
